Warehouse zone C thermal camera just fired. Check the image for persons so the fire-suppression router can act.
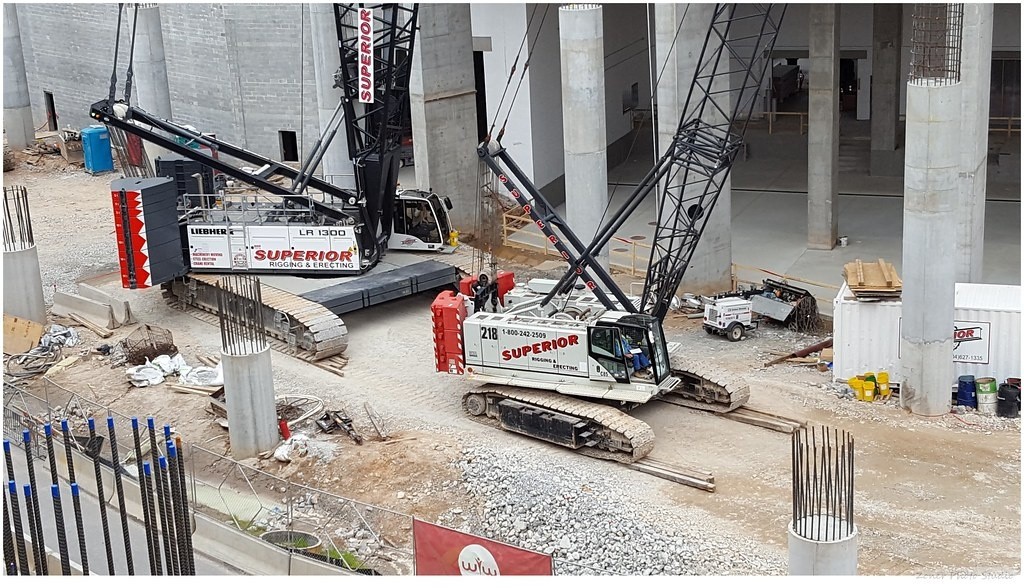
[615,330,652,379]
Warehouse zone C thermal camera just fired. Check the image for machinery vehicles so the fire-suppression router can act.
[432,7,809,494]
[91,2,464,377]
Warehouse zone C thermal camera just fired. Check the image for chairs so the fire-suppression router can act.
[410,208,435,237]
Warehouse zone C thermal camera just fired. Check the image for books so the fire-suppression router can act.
[629,347,642,354]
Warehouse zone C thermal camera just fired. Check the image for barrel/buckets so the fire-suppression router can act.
[848,371,889,403]
[956,375,1020,419]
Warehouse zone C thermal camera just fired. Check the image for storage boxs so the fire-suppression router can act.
[847,371,890,402]
[957,375,1021,419]
